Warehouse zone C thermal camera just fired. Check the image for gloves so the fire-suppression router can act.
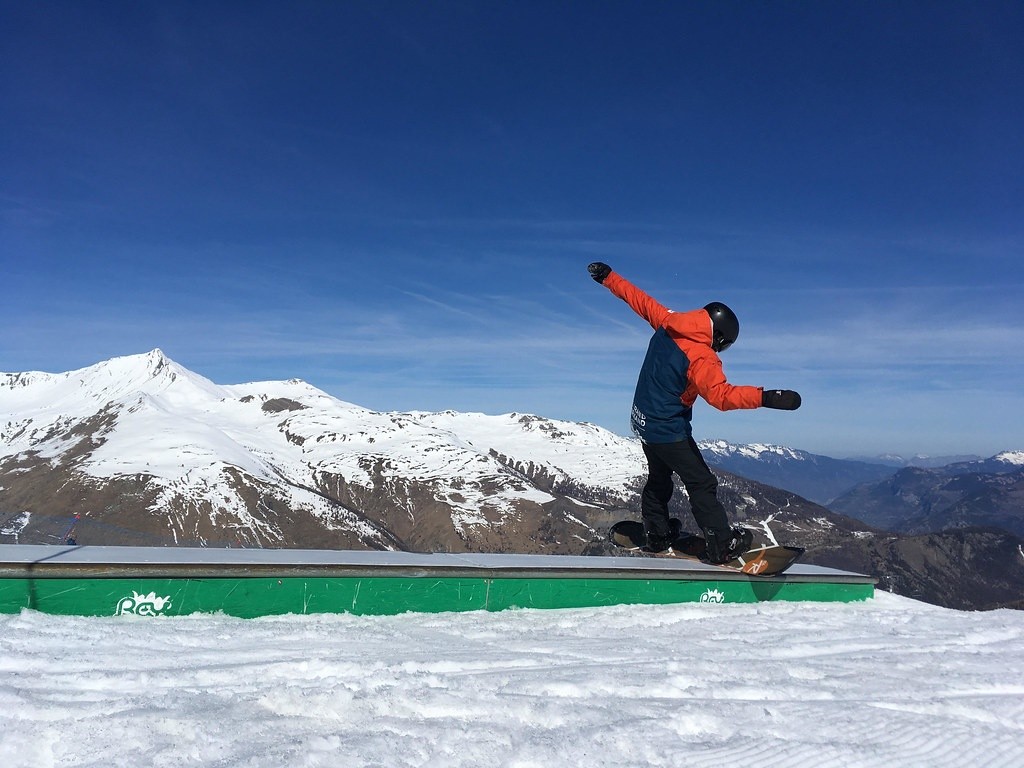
[762,389,802,410]
[587,261,612,284]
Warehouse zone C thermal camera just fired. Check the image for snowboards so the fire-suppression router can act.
[607,520,806,578]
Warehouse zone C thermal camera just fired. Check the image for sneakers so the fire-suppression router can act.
[645,518,682,552]
[701,528,753,564]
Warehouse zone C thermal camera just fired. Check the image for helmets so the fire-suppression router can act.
[703,302,740,352]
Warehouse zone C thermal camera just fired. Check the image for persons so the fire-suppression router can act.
[587,262,802,565]
